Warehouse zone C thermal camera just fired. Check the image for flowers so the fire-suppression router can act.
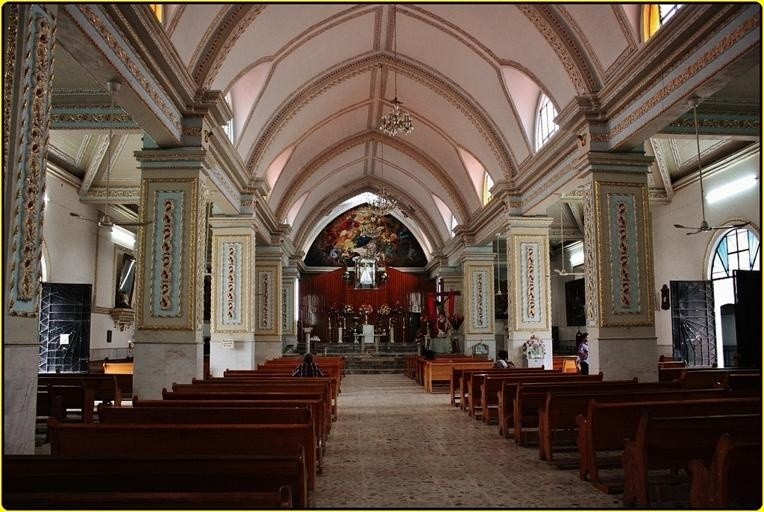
[335,314,345,321]
[322,209,397,286]
[359,304,373,316]
[327,302,339,316]
[341,303,353,315]
[376,304,392,318]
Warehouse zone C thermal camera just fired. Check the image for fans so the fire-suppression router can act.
[493,233,504,295]
[69,79,153,229]
[554,210,585,276]
[674,95,735,236]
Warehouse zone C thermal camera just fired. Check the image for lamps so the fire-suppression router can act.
[370,142,397,216]
[379,4,414,137]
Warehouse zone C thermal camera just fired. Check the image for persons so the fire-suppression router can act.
[578,333,589,375]
[292,354,323,377]
[493,351,515,368]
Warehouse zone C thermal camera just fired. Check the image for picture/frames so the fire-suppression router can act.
[355,257,376,291]
